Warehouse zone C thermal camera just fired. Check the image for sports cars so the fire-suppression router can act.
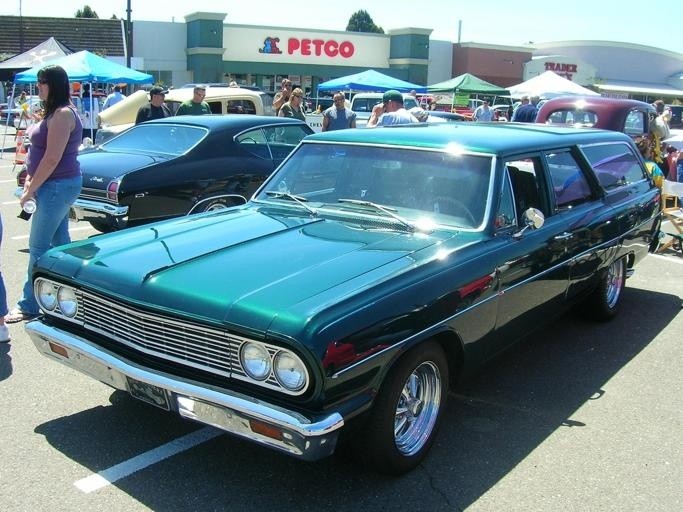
[14,112,345,228]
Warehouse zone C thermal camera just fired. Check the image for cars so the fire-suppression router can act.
[535,96,682,203]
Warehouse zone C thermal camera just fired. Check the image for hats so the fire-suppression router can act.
[150,87,169,94]
[383,90,402,102]
[292,87,304,97]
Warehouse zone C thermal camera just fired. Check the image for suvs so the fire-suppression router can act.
[21,119,669,475]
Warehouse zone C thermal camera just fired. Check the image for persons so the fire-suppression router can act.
[633,100,683,207]
[134,86,212,126]
[101,83,127,111]
[2,62,83,325]
[6,91,26,126]
[472,96,540,123]
[0,214,13,342]
[272,79,437,133]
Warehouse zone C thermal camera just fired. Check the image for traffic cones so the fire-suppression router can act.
[12,130,26,164]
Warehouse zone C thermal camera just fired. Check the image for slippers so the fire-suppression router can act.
[4,312,31,323]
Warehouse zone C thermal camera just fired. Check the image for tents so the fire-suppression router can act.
[316,69,428,111]
[424,74,514,115]
[497,70,601,105]
[0,36,76,82]
[6,50,154,145]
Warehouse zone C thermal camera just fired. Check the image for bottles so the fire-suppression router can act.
[676,160,683,184]
[22,196,37,215]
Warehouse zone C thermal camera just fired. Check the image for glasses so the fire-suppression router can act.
[38,79,48,84]
